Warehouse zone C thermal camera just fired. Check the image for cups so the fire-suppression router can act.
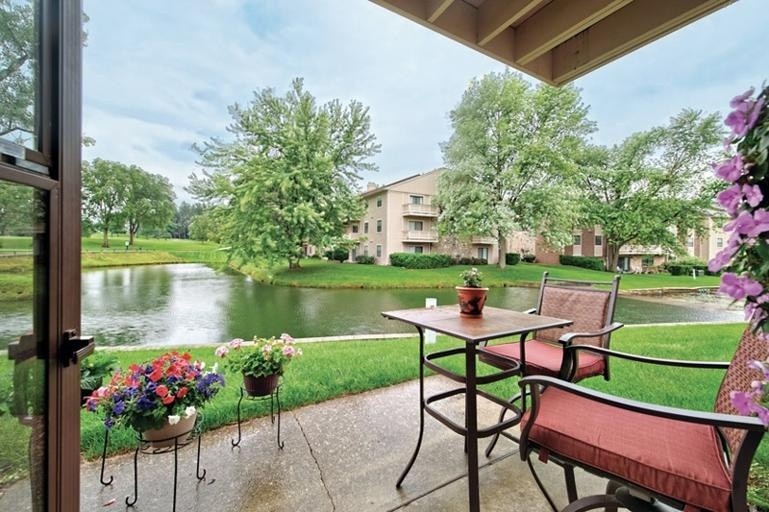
[425,297,437,311]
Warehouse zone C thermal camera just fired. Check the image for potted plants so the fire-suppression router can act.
[139,409,196,450]
[456,267,490,318]
[242,370,282,396]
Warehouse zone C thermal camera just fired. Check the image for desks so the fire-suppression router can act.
[382,300,574,511]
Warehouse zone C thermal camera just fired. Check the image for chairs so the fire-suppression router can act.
[518,308,769,512]
[479,270,621,459]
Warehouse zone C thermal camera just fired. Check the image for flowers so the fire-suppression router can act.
[708,85,769,422]
[216,334,295,378]
[85,348,225,432]
